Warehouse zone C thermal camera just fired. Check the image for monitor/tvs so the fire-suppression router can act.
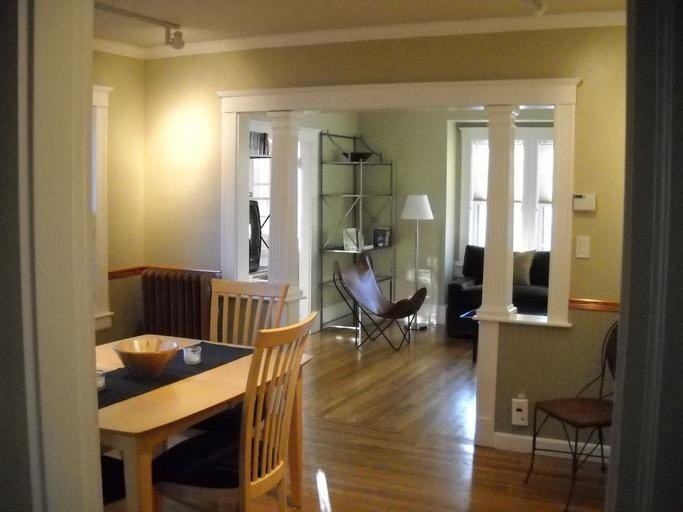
[248,199,263,273]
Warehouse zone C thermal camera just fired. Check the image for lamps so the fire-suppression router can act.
[400,194,434,330]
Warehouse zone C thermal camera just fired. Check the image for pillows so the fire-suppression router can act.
[513,251,536,285]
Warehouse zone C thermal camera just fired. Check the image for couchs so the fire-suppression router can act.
[447,245,550,338]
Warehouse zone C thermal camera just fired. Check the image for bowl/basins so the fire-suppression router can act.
[341,151,372,161]
[113,338,177,378]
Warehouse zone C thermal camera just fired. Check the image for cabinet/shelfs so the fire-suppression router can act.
[319,131,396,336]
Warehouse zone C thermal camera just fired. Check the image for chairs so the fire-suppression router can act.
[524,318,622,511]
[333,250,427,352]
[152,278,319,512]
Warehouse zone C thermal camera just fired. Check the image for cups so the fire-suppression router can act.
[184,346,201,366]
[342,228,357,251]
[97,370,105,391]
[373,227,391,249]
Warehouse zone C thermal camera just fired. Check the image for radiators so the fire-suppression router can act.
[138,268,224,341]
